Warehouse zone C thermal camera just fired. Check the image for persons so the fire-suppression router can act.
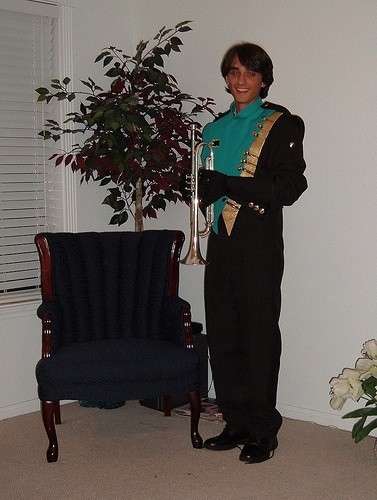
[175,41,308,463]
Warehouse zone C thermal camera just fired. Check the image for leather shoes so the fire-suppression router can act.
[204,423,246,450]
[240,434,279,462]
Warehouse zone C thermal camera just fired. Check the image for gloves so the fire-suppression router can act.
[199,169,275,217]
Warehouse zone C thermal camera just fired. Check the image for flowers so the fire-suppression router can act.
[328,339,377,443]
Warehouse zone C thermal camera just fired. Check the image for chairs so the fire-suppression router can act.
[34,231,205,463]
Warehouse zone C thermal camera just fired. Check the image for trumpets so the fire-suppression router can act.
[180,124,220,265]
